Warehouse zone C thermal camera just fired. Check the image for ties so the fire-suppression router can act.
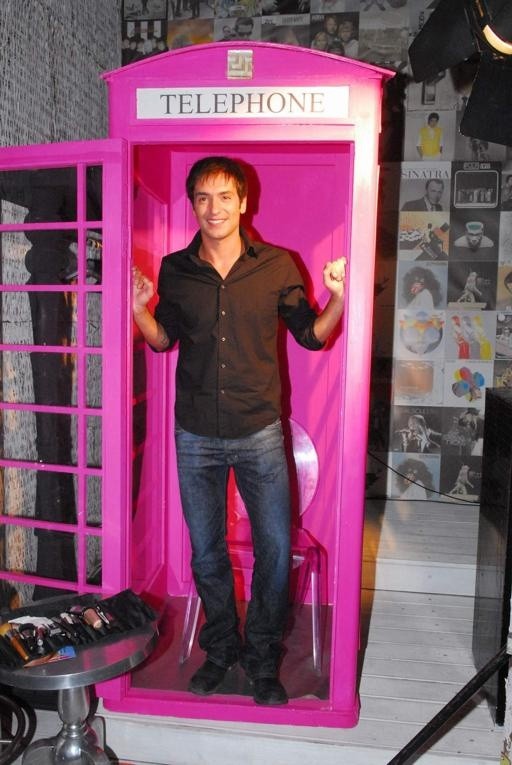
[430,205,432,209]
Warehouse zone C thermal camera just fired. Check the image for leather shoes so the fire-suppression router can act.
[252,676,287,706]
[188,657,237,695]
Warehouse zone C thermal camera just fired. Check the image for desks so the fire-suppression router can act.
[0,594,159,765]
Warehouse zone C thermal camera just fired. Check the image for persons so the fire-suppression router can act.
[120,0,359,66]
[127,156,347,709]
[400,112,511,498]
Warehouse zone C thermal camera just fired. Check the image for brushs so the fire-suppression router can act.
[0,592,155,668]
